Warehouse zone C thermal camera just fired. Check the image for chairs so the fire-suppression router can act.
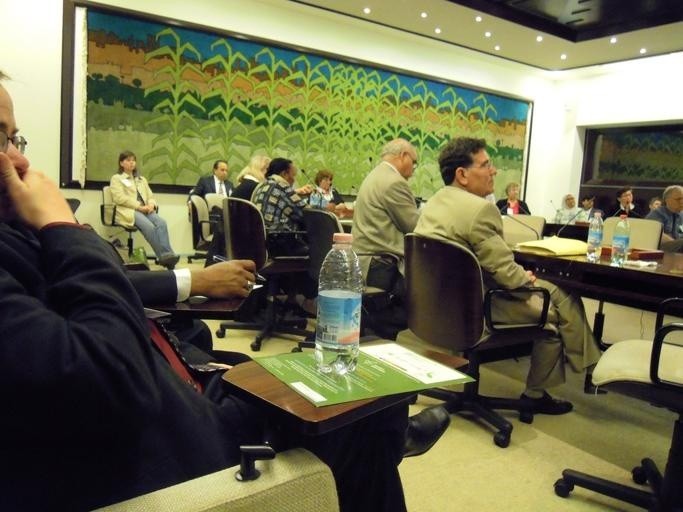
[552,291,683,509]
[395,227,536,449]
[187,194,221,264]
[499,213,546,245]
[216,196,314,352]
[291,201,399,351]
[203,193,226,210]
[590,216,665,350]
[97,184,159,265]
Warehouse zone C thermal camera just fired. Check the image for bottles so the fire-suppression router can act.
[611,214,631,264]
[137,247,146,263]
[310,190,319,209]
[314,231,362,375]
[585,212,604,260]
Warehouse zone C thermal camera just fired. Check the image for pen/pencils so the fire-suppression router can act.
[212,254,269,282]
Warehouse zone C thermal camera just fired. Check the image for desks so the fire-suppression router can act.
[339,219,682,350]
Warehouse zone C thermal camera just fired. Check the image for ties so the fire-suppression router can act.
[218,182,224,195]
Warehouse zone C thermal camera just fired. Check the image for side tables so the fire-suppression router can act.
[223,343,479,428]
[146,286,254,319]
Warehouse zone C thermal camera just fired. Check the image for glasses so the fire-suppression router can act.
[464,159,494,171]
[407,152,419,170]
[1,131,26,155]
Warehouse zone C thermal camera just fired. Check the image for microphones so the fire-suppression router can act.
[494,195,639,241]
[300,168,319,194]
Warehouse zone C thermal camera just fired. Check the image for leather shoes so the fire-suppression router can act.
[404,406,452,457]
[520,392,575,416]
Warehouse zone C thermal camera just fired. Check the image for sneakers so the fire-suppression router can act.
[156,255,181,270]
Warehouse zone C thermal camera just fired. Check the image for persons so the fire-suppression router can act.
[351,138,424,344]
[412,135,609,414]
[497,183,531,215]
[126,261,257,373]
[1,71,450,510]
[192,152,349,300]
[108,150,179,269]
[555,185,682,252]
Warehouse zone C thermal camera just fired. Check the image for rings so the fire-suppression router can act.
[247,281,254,290]
